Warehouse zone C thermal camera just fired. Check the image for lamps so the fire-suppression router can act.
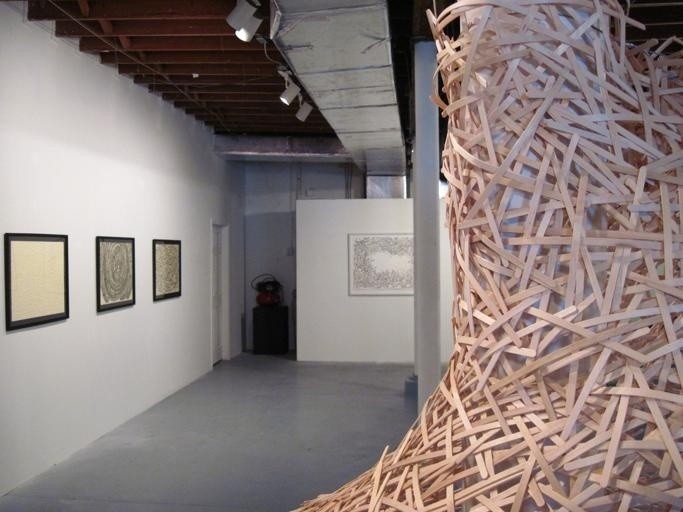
[226,1,314,124]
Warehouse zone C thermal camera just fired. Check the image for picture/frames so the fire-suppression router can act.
[345,232,414,297]
[150,238,182,301]
[93,235,138,313]
[3,232,71,332]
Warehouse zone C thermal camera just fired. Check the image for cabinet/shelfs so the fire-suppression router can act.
[252,306,288,355]
